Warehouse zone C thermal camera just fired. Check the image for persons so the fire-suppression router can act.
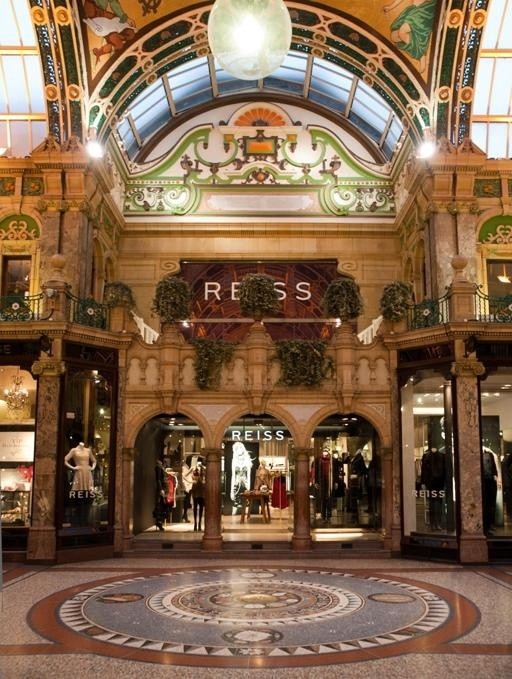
[180,459,194,522]
[482,447,499,532]
[420,447,448,530]
[253,459,273,515]
[183,461,207,532]
[309,448,370,526]
[64,440,99,491]
[230,441,257,516]
[151,459,171,533]
[162,467,180,523]
